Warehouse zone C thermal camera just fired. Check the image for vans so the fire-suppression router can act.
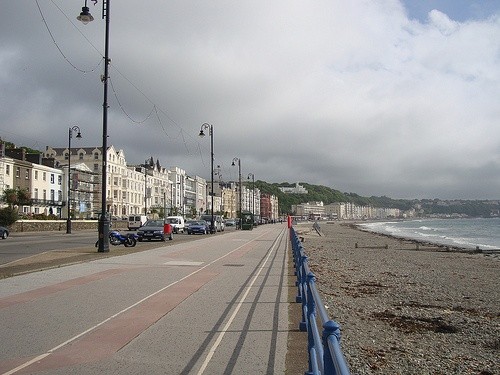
[167,216,185,234]
[127,215,147,230]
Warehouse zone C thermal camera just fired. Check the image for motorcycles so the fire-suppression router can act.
[95,222,138,247]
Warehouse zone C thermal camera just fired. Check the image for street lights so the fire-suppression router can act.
[170,183,181,216]
[247,172,255,227]
[198,122,214,234]
[231,156,242,231]
[75,0,111,253]
[66,126,83,234]
[144,161,154,216]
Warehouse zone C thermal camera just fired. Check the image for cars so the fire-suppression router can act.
[225,220,235,226]
[0,225,9,240]
[183,214,224,234]
[137,219,174,242]
